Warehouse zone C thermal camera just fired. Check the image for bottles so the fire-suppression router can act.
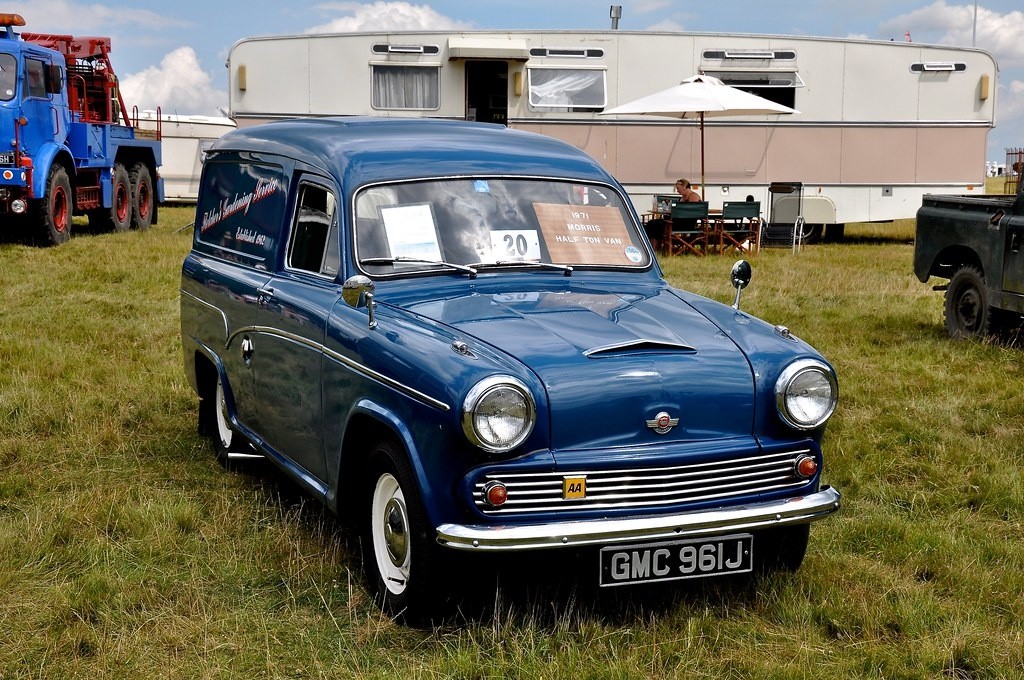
[662,201,666,210]
[652,195,657,210]
[668,200,673,210]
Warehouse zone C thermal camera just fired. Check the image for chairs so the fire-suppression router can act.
[757,182,806,254]
[714,201,761,257]
[661,201,709,258]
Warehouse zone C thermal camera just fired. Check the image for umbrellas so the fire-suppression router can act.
[597,73,803,202]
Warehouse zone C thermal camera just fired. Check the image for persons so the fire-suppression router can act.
[674,179,702,200]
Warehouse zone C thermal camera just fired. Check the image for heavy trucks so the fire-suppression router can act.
[0,14,164,248]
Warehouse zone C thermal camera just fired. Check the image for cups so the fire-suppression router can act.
[658,205,663,212]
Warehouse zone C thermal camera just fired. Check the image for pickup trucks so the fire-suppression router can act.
[912,147,1023,346]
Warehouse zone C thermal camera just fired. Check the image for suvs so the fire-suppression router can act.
[179,116,843,616]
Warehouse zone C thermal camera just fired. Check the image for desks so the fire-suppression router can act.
[648,210,723,213]
